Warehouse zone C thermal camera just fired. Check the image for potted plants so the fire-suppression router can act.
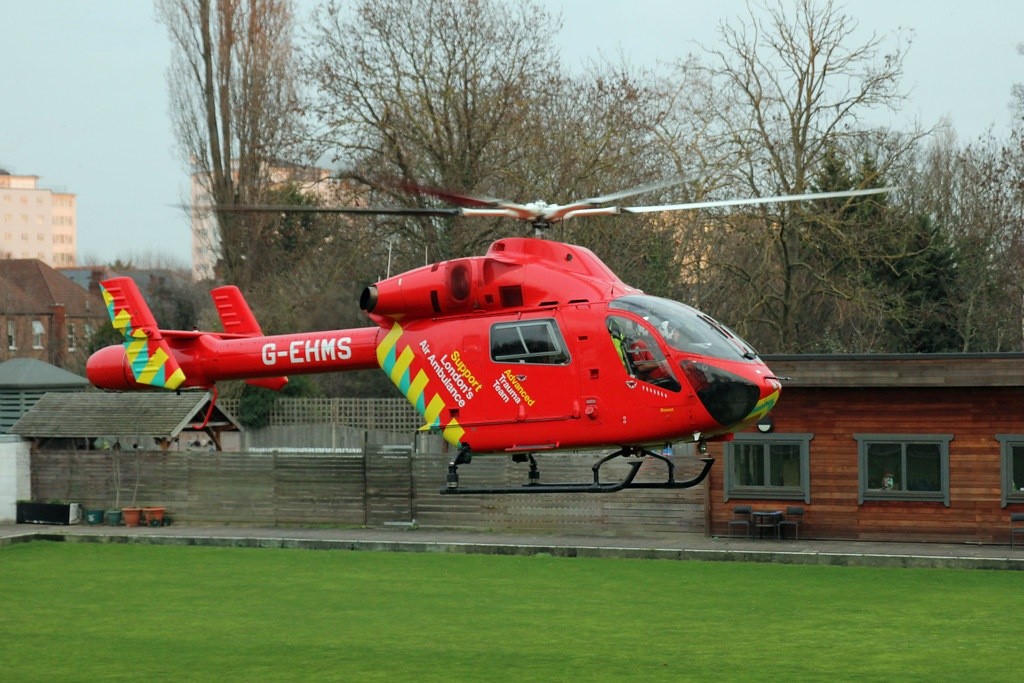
[122,505,142,527]
[107,508,122,525]
[14,496,80,525]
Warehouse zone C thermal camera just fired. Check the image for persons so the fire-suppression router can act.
[633,316,681,379]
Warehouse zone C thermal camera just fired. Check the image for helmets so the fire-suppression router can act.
[638,316,661,336]
[657,321,683,339]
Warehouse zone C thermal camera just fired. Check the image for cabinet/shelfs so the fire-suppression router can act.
[751,510,782,541]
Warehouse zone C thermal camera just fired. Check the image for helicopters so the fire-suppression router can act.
[83,152,914,495]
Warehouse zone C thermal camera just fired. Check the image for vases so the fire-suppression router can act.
[144,504,163,528]
[86,508,105,525]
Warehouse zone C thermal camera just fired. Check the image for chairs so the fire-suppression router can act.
[778,506,803,539]
[728,506,752,538]
[1010,513,1024,550]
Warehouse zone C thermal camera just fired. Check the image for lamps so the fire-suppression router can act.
[757,416,774,433]
